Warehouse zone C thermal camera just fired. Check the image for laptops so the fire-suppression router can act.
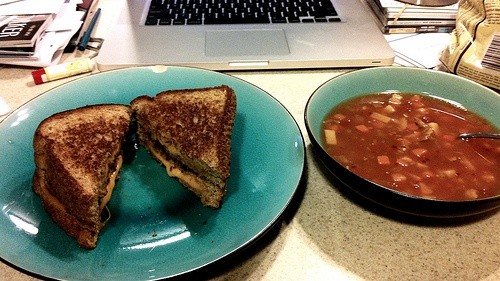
[95,0,397,68]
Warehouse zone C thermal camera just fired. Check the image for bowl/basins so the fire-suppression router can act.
[303,66,500,221]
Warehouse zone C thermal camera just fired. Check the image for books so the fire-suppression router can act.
[369,0,460,36]
[0,0,86,68]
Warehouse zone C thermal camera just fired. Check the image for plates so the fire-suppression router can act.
[1,65,306,281]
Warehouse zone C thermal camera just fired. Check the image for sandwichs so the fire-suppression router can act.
[129,86,236,208]
[30,103,136,248]
